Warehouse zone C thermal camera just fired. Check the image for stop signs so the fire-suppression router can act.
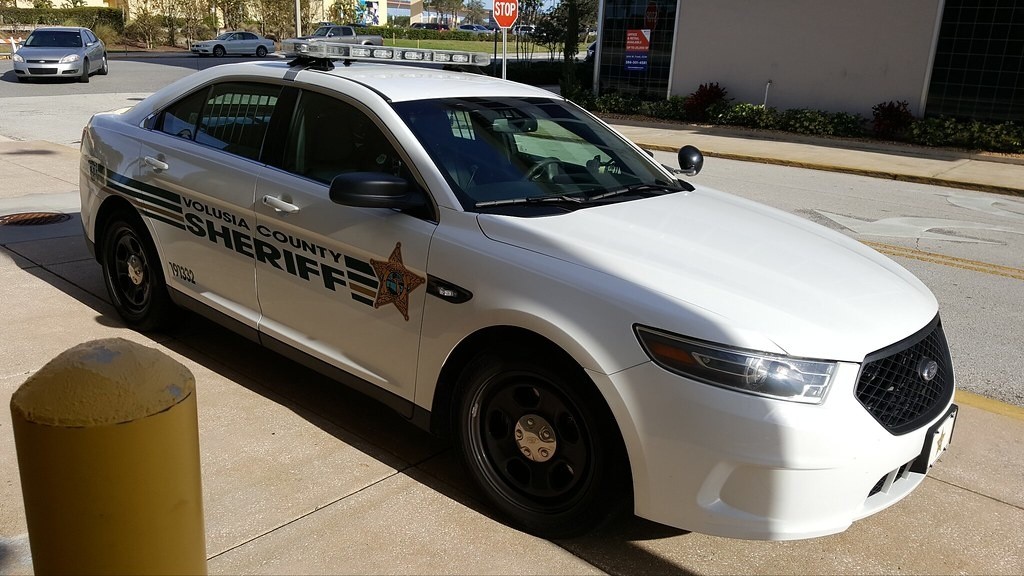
[493,0,519,29]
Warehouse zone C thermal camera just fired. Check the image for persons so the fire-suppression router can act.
[416,111,475,186]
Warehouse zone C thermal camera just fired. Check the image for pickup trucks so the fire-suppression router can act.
[297,24,383,47]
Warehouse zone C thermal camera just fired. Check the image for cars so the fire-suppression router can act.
[190,30,277,58]
[458,23,494,37]
[409,22,451,31]
[511,24,546,39]
[76,36,959,542]
[584,40,597,62]
[11,25,109,83]
